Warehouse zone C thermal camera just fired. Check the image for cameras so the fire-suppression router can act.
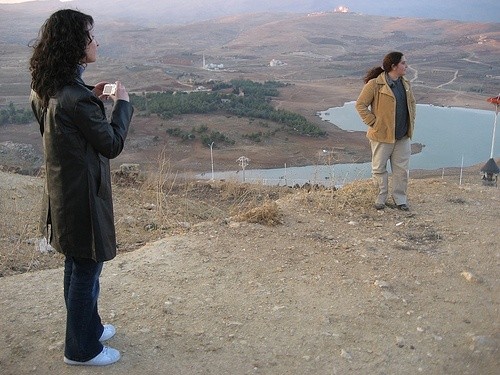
[102,84,117,96]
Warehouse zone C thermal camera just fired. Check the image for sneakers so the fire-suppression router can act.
[64,346,120,366]
[99,324,115,341]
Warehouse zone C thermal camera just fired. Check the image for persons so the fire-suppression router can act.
[30,9,134,366]
[355,52,416,211]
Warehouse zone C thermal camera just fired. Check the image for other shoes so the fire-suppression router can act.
[376,203,384,209]
[399,204,408,211]
